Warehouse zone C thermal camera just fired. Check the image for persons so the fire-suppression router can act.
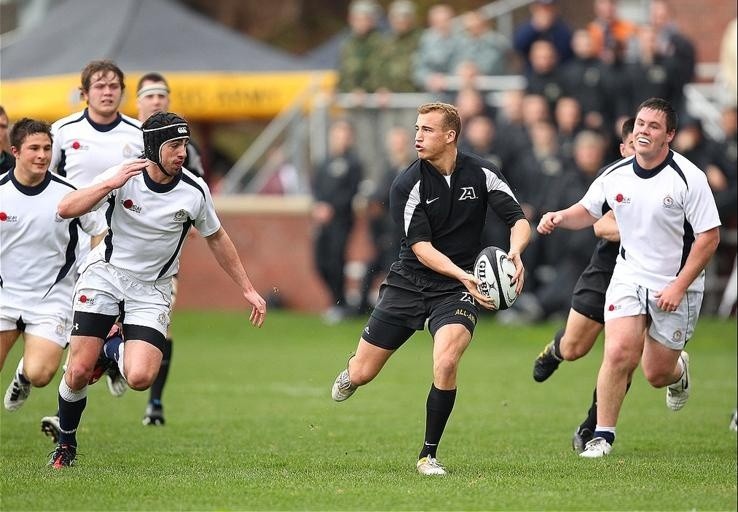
[536,98,722,457]
[532,115,636,453]
[331,102,531,475]
[260,145,296,194]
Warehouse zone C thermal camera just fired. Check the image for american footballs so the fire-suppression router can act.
[474,246,518,310]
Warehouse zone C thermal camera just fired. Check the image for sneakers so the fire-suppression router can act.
[532,340,563,382]
[580,437,613,458]
[665,350,690,411]
[46,443,77,468]
[572,425,595,450]
[40,412,60,444]
[142,400,167,426]
[88,332,122,385]
[107,365,128,397]
[415,456,447,474]
[3,357,33,412]
[332,368,358,402]
